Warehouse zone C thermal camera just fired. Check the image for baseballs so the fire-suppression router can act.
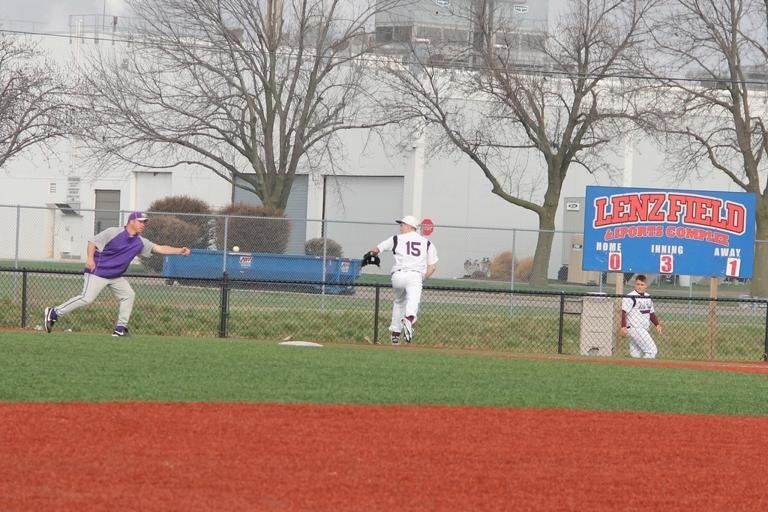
[233,246,240,252]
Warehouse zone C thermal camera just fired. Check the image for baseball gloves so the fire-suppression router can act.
[361,251,381,268]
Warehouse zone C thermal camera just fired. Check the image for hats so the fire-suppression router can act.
[395,216,418,231]
[128,212,149,222]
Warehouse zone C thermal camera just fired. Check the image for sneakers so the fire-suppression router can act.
[391,334,400,346]
[44,307,56,333]
[111,330,128,336]
[401,318,414,344]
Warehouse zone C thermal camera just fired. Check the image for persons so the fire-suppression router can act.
[40,208,189,335]
[620,274,662,361]
[361,216,440,348]
